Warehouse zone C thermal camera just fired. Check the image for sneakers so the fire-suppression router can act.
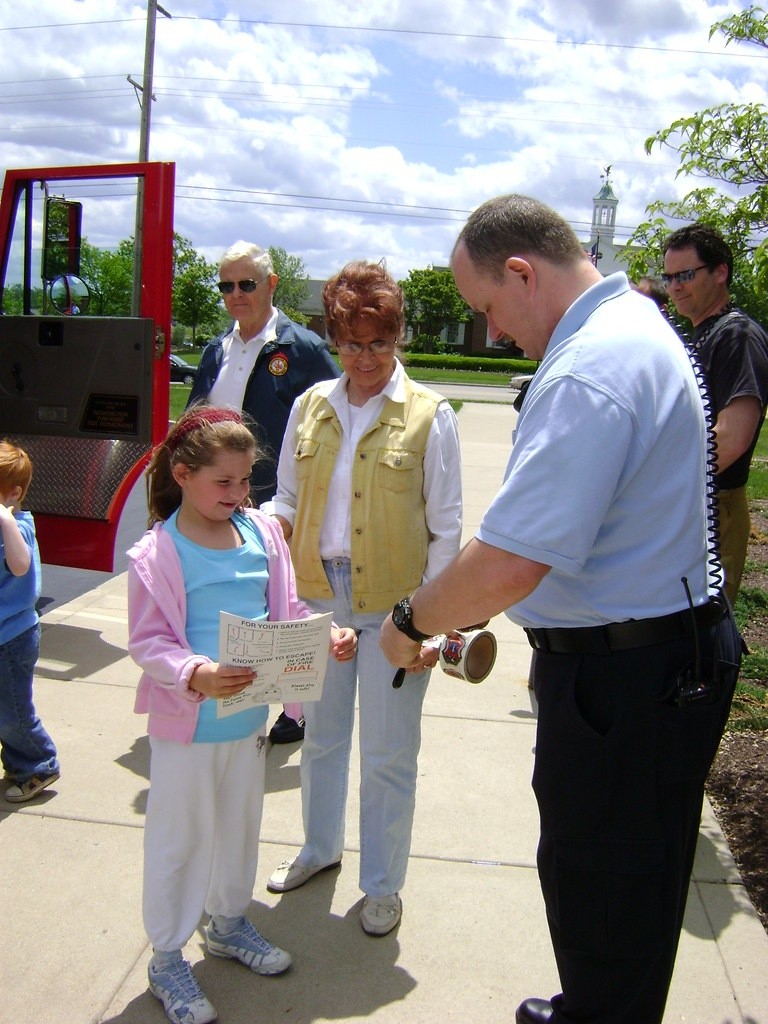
[207,916,292,975]
[5,772,60,803]
[147,958,217,1024]
[359,891,400,934]
[267,852,342,892]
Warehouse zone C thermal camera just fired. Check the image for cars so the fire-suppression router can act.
[509,376,532,390]
[170,353,199,386]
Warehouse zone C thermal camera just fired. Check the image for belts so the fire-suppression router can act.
[523,587,727,655]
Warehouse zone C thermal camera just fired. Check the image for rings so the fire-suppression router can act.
[423,665,431,670]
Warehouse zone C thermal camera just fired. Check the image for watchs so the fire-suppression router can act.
[391,597,435,643]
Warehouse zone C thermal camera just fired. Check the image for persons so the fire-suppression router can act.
[259,260,463,936]
[184,240,344,745]
[379,194,750,1024]
[126,404,358,1024]
[0,440,61,803]
[631,224,768,614]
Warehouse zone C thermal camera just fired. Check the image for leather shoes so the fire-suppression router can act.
[515,998,558,1024]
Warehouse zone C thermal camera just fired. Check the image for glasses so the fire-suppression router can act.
[661,264,710,286]
[336,334,397,356]
[217,275,269,294]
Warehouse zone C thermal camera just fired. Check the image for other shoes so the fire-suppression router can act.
[269,710,305,745]
